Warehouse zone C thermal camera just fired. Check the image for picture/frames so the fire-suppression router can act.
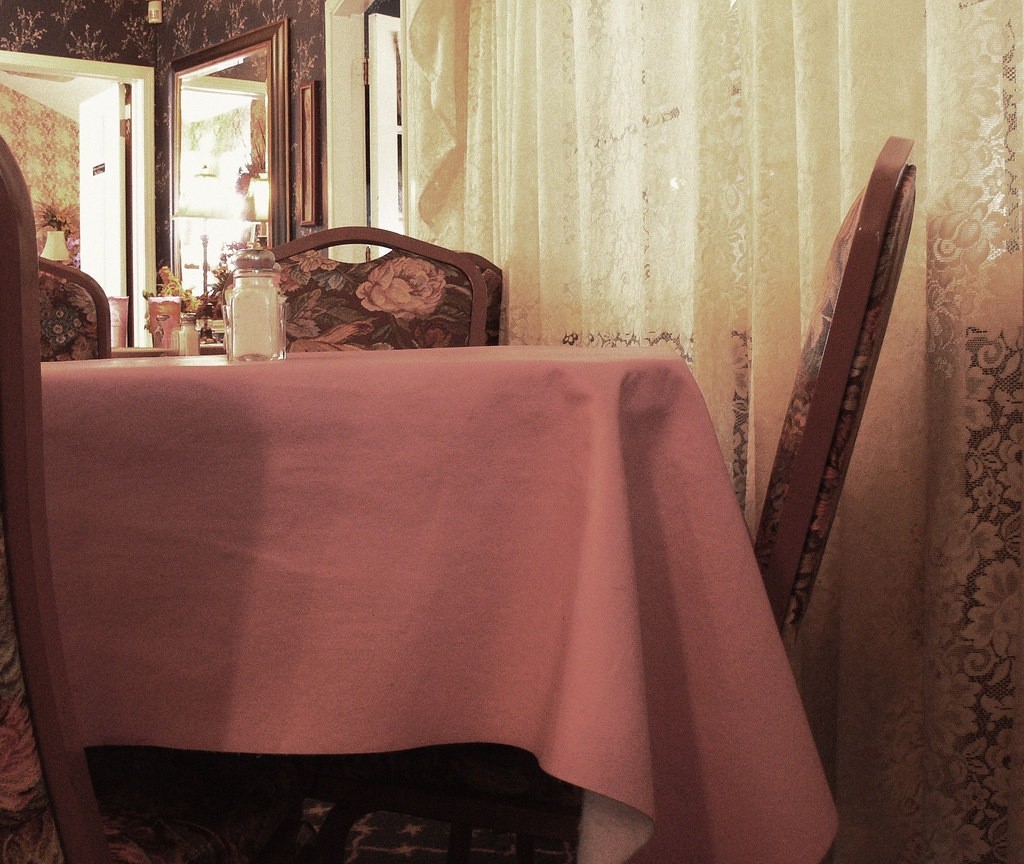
[298,80,318,228]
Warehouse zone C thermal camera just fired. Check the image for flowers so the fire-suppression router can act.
[67,238,79,269]
[142,239,247,335]
[33,199,79,230]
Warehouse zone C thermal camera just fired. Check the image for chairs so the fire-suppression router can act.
[220,227,502,353]
[2,134,113,864]
[37,257,110,363]
[755,137,917,660]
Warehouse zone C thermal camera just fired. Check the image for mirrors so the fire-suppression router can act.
[169,18,291,334]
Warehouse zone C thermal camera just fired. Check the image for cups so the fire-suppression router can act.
[148,296,181,348]
[107,296,129,348]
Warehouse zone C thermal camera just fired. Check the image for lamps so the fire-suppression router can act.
[40,231,70,260]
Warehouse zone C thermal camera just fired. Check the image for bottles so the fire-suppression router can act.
[176,314,199,356]
[224,248,287,361]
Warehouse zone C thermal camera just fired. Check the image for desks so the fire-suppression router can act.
[39,348,840,864]
